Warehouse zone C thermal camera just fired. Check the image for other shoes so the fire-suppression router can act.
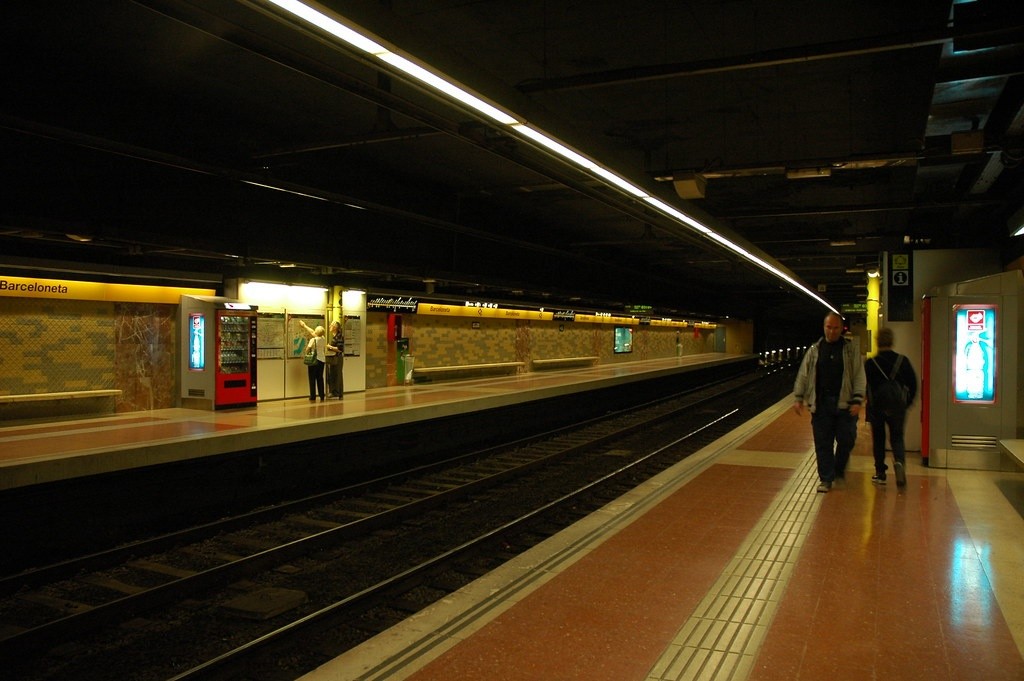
[309,397,316,400]
[321,393,324,400]
[872,475,887,484]
[893,461,906,486]
[817,481,831,491]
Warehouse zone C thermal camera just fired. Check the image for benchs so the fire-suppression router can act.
[533,355,600,372]
[0,388,123,413]
[414,361,526,375]
[998,438,1024,470]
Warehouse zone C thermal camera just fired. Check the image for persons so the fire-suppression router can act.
[298,320,326,403]
[863,327,918,488]
[327,321,344,400]
[792,313,866,493]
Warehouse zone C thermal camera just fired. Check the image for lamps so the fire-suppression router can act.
[672,171,706,199]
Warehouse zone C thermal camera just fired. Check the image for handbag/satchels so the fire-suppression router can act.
[325,350,338,365]
[871,354,907,418]
[304,339,318,366]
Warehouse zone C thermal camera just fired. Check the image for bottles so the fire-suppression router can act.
[219,317,249,373]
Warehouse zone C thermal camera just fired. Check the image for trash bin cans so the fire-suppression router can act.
[677,344,683,357]
[404,356,415,386]
